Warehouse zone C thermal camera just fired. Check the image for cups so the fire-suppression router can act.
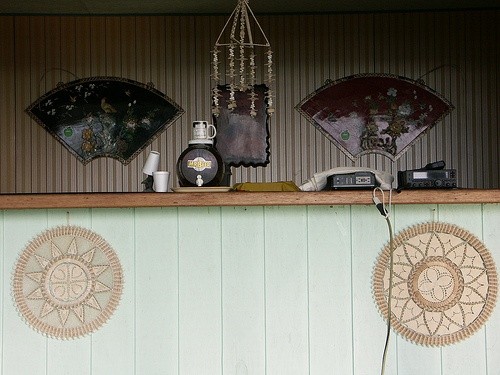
[193,121,216,140]
[152,171,169,192]
[142,151,160,176]
